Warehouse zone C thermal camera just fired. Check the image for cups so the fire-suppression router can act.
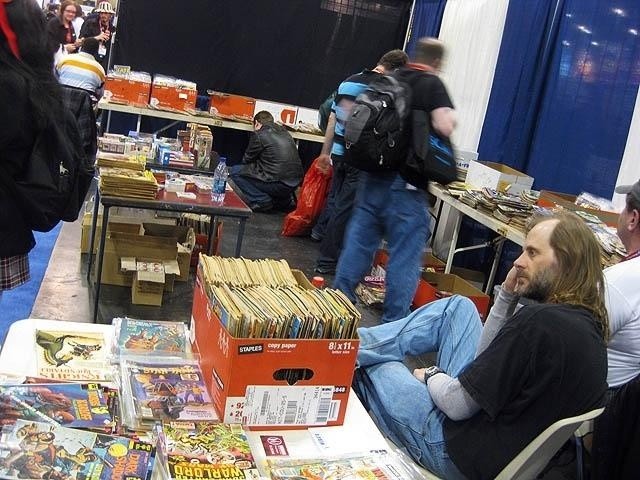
[135,93,148,113]
[114,66,131,75]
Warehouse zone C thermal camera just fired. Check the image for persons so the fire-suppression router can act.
[0,0,116,297]
[332,36,460,325]
[311,50,410,273]
[231,111,304,214]
[573,178,640,450]
[352,211,610,480]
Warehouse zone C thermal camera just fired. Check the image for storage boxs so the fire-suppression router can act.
[465,159,535,199]
[104,69,323,134]
[414,273,490,323]
[372,248,450,275]
[535,187,621,227]
[77,209,224,308]
[183,264,361,428]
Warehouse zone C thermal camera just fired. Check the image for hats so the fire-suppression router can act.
[94,2,116,14]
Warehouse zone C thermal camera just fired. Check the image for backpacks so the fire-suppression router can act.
[316,90,338,134]
[345,69,435,171]
[5,55,99,232]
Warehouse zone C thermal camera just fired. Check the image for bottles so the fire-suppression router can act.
[211,158,229,202]
[310,276,324,292]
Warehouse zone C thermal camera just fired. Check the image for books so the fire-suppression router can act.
[92,121,217,236]
[438,168,626,274]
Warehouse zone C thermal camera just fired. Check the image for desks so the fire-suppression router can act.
[1,317,414,480]
[89,173,253,322]
[94,96,330,158]
[428,180,626,295]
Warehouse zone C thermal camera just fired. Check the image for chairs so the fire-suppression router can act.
[496,407,609,479]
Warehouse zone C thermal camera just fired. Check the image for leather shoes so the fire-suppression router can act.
[249,202,273,212]
[285,199,296,213]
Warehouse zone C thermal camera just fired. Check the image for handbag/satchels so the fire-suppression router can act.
[408,107,456,186]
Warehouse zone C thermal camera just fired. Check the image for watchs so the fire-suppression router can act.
[424,366,446,386]
